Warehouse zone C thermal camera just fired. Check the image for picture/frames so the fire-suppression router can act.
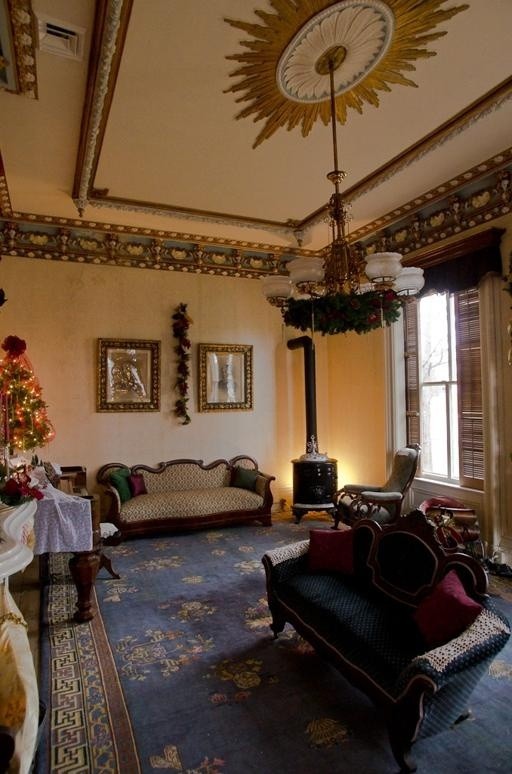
[198,343,251,411]
[96,337,160,412]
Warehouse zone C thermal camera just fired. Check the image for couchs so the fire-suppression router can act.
[97,455,275,541]
[260,510,511,773]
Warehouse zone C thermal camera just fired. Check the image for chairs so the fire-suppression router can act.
[331,444,421,530]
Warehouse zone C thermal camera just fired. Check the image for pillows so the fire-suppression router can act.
[126,472,148,497]
[307,528,353,570]
[107,467,131,504]
[411,568,484,643]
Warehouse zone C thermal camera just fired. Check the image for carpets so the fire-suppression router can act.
[31,510,511,774]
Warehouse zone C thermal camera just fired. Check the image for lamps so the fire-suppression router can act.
[262,56,425,351]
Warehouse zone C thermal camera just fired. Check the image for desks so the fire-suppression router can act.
[0,495,100,622]
